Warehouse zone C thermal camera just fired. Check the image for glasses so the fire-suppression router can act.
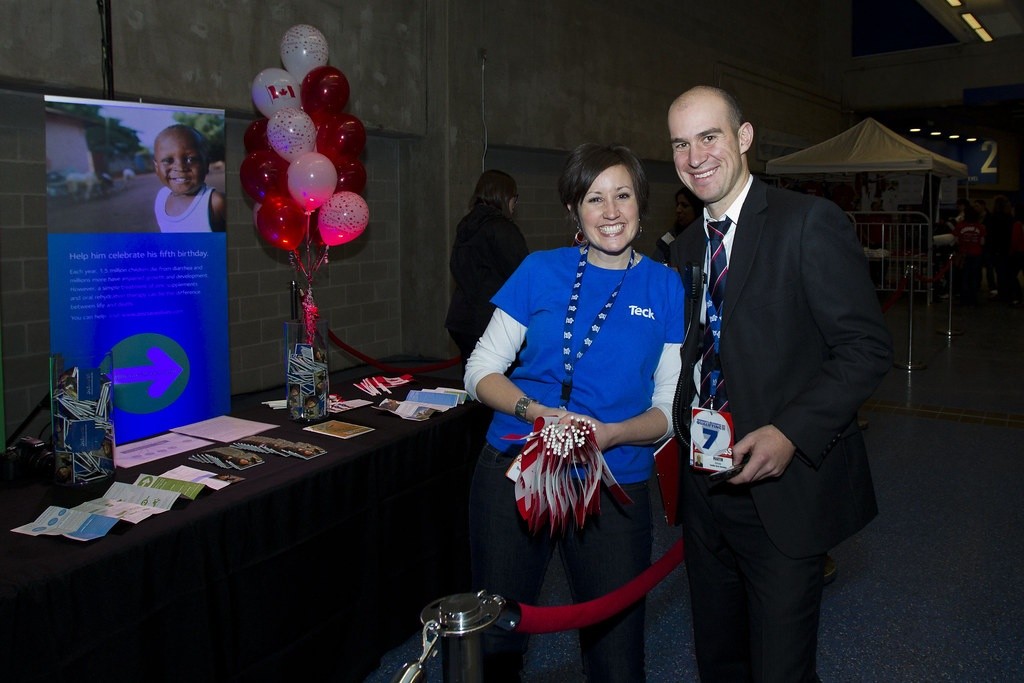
[511,194,519,204]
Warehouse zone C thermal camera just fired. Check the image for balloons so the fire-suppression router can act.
[310,208,326,246]
[267,108,316,162]
[317,192,369,246]
[280,24,330,85]
[316,114,367,167]
[252,68,301,117]
[286,151,337,211]
[243,119,268,151]
[239,151,289,200]
[332,155,367,195]
[301,65,350,125]
[257,198,304,251]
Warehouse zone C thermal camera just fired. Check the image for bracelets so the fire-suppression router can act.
[516,395,539,423]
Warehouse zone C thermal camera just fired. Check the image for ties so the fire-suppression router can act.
[699,217,733,411]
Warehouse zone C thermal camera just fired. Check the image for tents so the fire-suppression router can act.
[764,117,971,307]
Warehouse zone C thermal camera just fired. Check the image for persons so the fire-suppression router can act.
[695,455,703,467]
[666,85,896,683]
[444,169,530,382]
[152,123,225,232]
[463,140,685,683]
[649,186,704,264]
[950,195,1024,309]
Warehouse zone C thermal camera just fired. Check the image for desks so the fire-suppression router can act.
[0,351,479,683]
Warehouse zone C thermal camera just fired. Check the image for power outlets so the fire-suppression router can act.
[477,47,487,66]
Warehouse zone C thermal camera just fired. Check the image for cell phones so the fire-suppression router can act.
[696,460,749,498]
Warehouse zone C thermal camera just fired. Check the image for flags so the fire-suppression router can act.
[354,374,414,389]
[499,415,635,537]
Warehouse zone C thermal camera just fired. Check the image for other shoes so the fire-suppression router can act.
[990,289,998,295]
[822,557,838,585]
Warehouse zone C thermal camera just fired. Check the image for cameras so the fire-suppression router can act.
[0,435,56,486]
